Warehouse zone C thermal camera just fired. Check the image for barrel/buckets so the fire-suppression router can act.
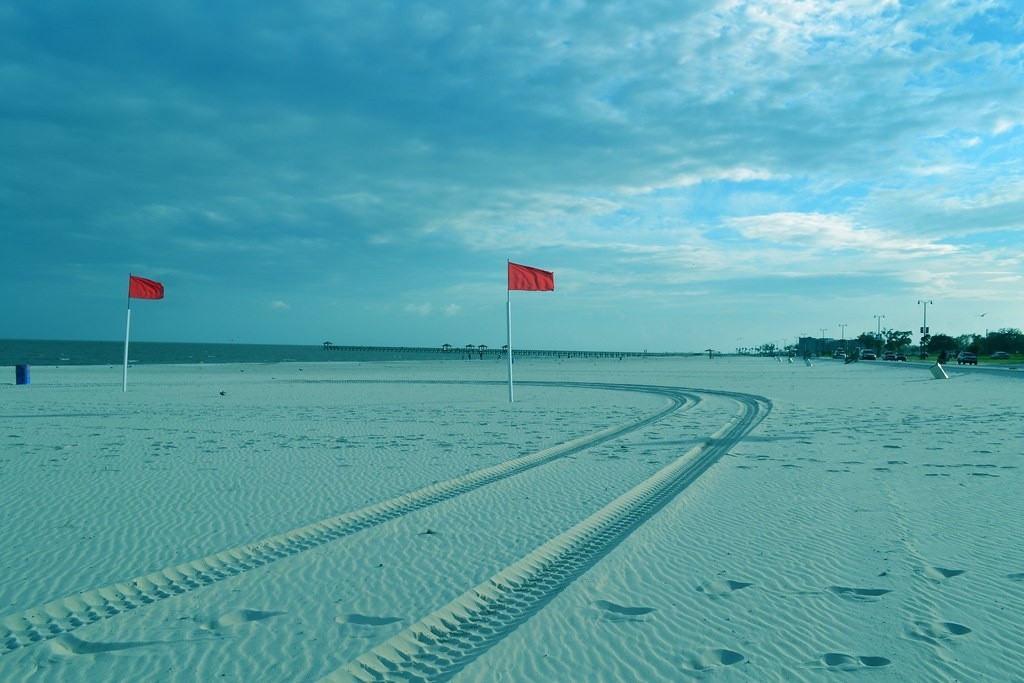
[16,363,30,385]
[928,363,948,379]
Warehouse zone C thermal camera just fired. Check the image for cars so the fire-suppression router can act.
[919,352,929,357]
[896,353,907,362]
[991,351,1009,358]
[957,352,977,365]
[882,351,896,361]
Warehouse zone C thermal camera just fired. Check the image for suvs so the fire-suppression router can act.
[835,350,846,359]
[859,349,876,360]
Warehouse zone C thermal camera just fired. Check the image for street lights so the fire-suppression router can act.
[873,315,885,339]
[820,329,827,339]
[839,324,847,339]
[918,300,932,359]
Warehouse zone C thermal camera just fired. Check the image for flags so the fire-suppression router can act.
[129,276,164,300]
[508,261,554,291]
